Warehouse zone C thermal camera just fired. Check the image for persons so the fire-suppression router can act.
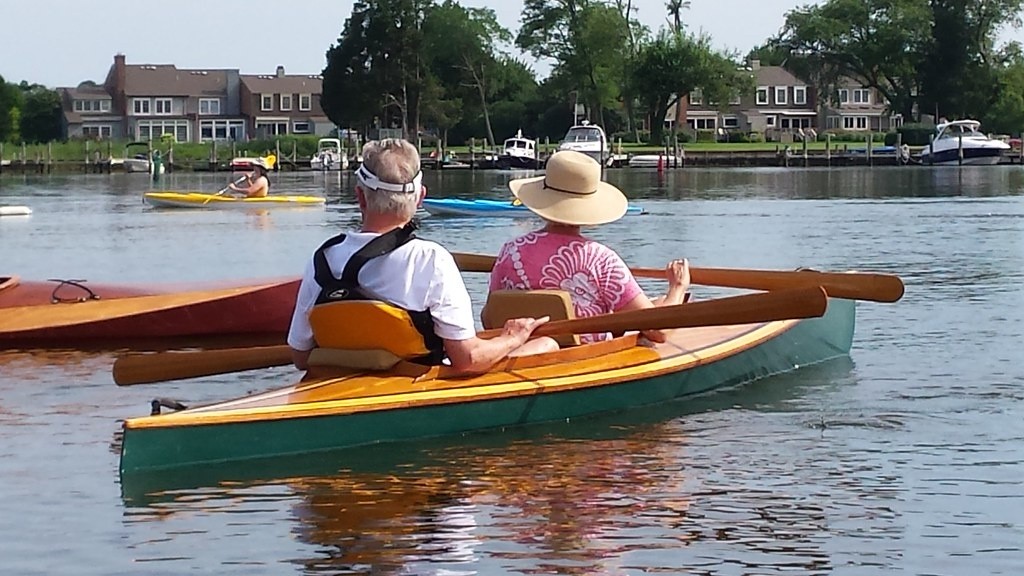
[287,138,560,373]
[228,157,275,197]
[489,151,690,344]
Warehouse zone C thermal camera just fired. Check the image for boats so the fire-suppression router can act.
[309,138,349,170]
[123,142,166,174]
[141,191,327,207]
[492,129,545,170]
[556,119,611,164]
[921,119,1012,166]
[0,272,304,343]
[628,154,683,169]
[115,266,856,501]
[229,154,276,172]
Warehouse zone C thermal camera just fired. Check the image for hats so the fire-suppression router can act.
[354,162,423,193]
[508,150,629,226]
[252,157,273,169]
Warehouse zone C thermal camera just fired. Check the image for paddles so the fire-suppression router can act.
[110,285,827,386]
[203,155,277,205]
[448,251,906,307]
